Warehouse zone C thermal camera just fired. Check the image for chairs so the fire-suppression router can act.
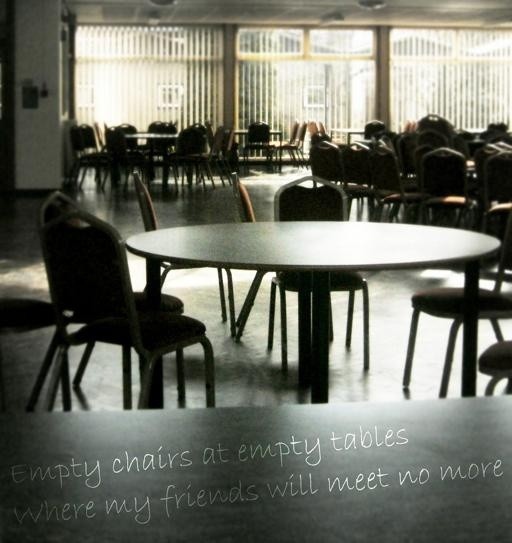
[3,113,511,415]
